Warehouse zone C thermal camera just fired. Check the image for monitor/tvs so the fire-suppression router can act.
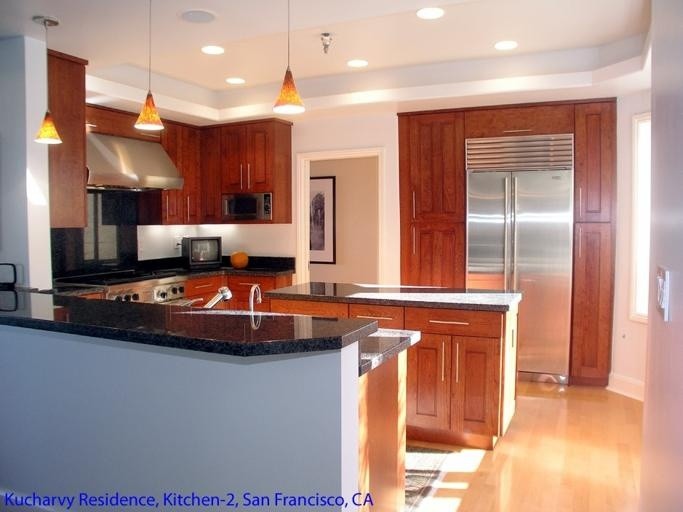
[181,236,222,269]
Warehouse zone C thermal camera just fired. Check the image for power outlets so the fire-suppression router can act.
[173,235,181,249]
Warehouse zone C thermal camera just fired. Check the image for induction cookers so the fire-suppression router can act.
[55,268,186,303]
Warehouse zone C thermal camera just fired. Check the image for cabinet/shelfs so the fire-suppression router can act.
[190,273,225,310]
[200,124,221,223]
[45,48,91,233]
[272,298,348,321]
[162,122,202,225]
[466,101,574,139]
[348,301,404,330]
[569,96,617,386]
[396,109,465,290]
[402,301,519,452]
[226,272,292,314]
[82,102,162,144]
[221,120,292,224]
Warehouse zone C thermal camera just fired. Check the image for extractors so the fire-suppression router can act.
[85,132,182,192]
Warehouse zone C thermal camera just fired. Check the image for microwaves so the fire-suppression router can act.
[222,192,272,222]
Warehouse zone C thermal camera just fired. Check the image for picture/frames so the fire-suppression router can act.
[309,175,336,265]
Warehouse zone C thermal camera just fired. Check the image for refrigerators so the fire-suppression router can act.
[465,135,573,388]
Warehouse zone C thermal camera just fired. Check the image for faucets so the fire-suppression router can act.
[247,284,263,312]
[250,316,262,330]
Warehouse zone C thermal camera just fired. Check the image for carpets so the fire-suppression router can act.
[404,438,457,512]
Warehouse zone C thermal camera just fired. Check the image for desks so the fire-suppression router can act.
[0,285,380,512]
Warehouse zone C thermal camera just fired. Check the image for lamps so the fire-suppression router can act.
[133,0,165,131]
[272,0,307,116]
[30,14,62,147]
